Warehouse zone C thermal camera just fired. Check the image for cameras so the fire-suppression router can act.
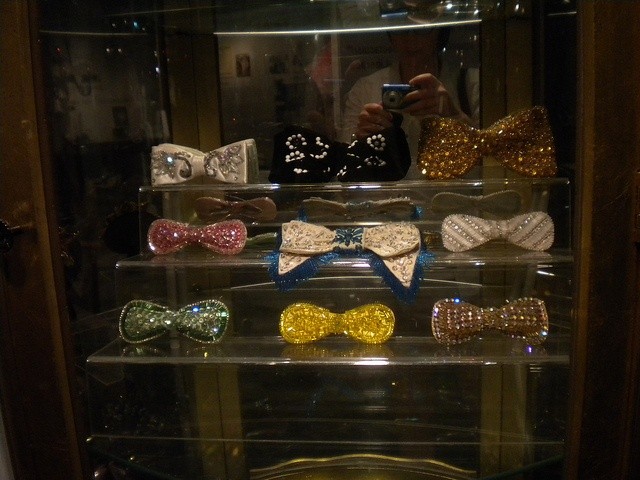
[381,84,428,109]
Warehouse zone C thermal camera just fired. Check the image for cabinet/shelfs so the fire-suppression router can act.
[84,165,572,450]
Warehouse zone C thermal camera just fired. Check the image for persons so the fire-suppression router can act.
[339,22,482,152]
